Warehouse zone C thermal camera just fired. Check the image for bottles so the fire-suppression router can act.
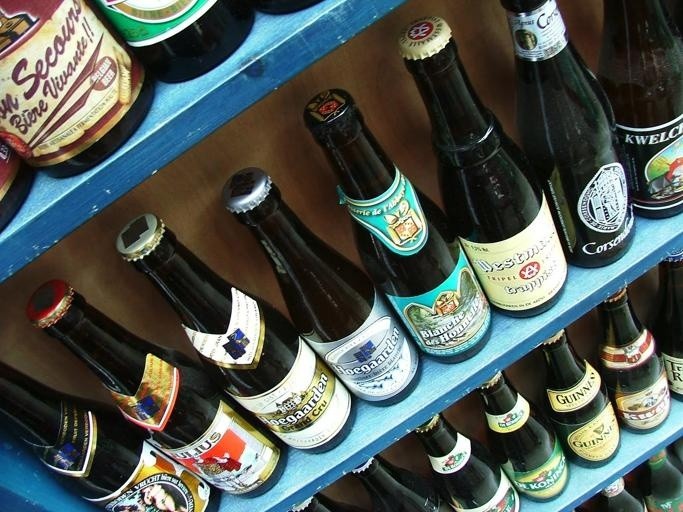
[0,0,683,512]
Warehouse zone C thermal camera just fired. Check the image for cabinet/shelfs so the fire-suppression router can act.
[0,0,683,512]
